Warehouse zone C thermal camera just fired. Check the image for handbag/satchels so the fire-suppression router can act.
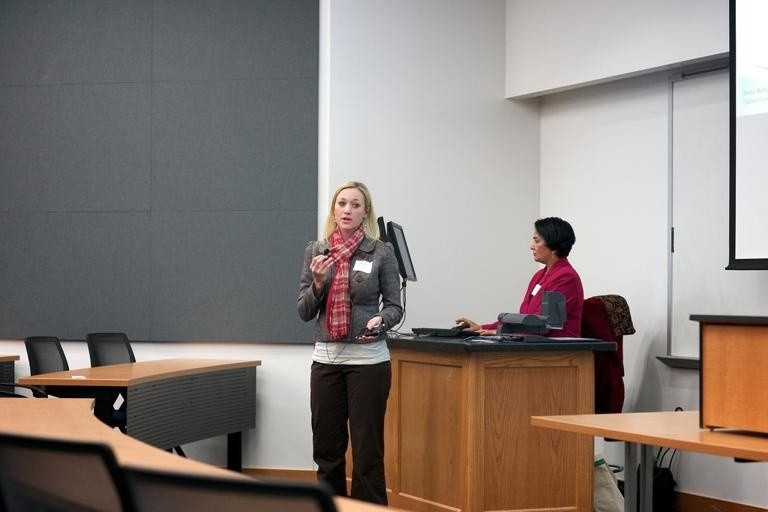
[638,463,676,512]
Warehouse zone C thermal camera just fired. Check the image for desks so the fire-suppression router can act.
[19,358,264,470]
[0,397,387,511]
[528,409,768,509]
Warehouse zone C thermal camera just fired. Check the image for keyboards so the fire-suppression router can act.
[412,328,480,337]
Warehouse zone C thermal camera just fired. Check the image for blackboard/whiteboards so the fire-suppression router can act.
[657,67,768,369]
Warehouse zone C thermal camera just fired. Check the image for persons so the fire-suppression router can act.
[296,182,403,508]
[456,218,584,339]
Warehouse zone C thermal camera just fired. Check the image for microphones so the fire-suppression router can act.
[323,248,329,254]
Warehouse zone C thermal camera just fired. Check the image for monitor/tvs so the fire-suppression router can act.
[388,220,417,282]
[377,216,388,242]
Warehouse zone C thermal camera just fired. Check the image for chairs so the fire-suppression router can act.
[86,332,188,457]
[2,354,21,393]
[23,335,69,376]
[578,297,629,415]
